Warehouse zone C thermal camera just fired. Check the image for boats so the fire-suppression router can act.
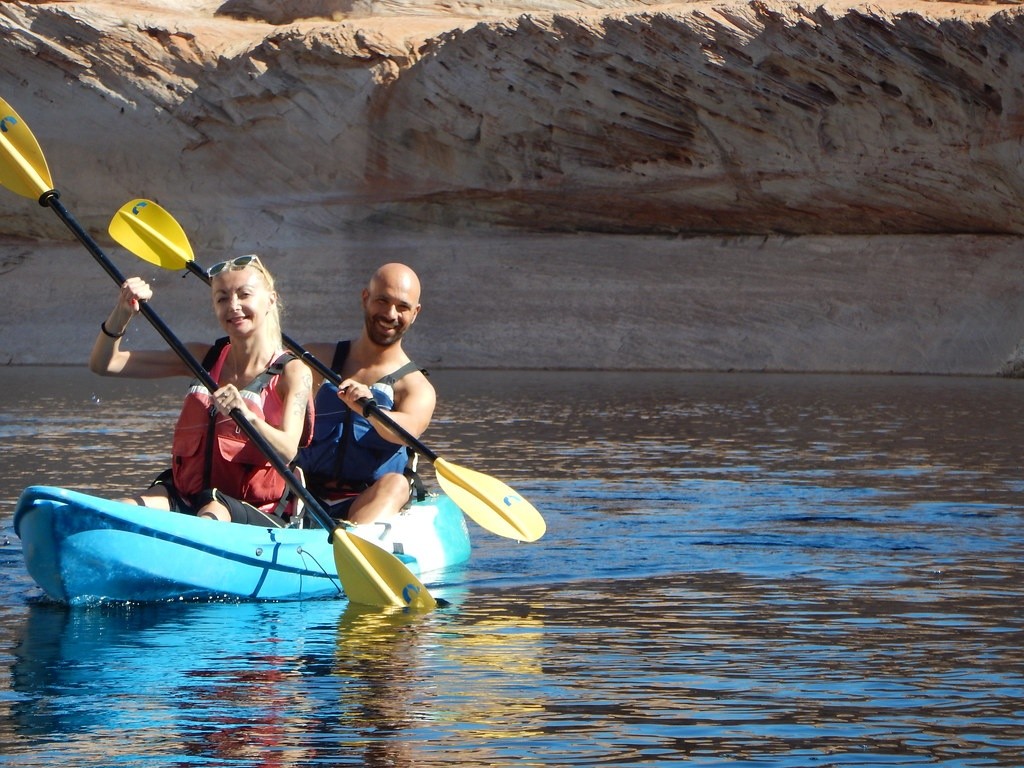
[13,485,472,611]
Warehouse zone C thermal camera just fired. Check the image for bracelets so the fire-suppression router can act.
[100,321,126,337]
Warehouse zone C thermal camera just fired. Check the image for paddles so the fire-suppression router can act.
[107,196,547,546]
[0,95,440,616]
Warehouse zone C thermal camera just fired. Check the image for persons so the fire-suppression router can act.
[89,251,315,529]
[292,262,437,529]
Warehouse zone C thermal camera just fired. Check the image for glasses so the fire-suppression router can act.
[206,254,271,285]
